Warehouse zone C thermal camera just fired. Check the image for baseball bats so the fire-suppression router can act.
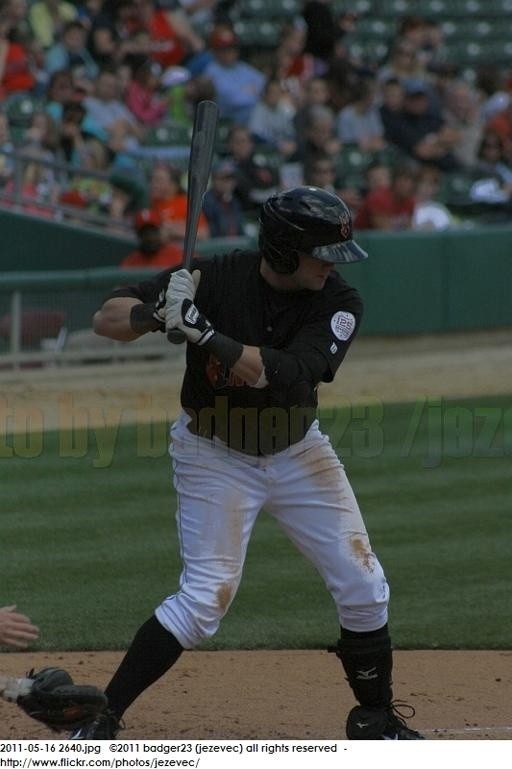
[169,100,218,343]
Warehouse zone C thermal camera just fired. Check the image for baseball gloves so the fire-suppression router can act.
[2,666,107,730]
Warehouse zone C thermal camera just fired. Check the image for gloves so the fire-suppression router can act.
[128,268,244,373]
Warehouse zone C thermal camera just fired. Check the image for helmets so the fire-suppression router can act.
[256,185,369,275]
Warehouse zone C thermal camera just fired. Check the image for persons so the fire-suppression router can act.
[0,601,41,650]
[0,0,511,364]
[72,185,424,740]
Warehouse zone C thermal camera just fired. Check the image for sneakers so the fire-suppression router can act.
[69,711,119,740]
[344,703,426,740]
